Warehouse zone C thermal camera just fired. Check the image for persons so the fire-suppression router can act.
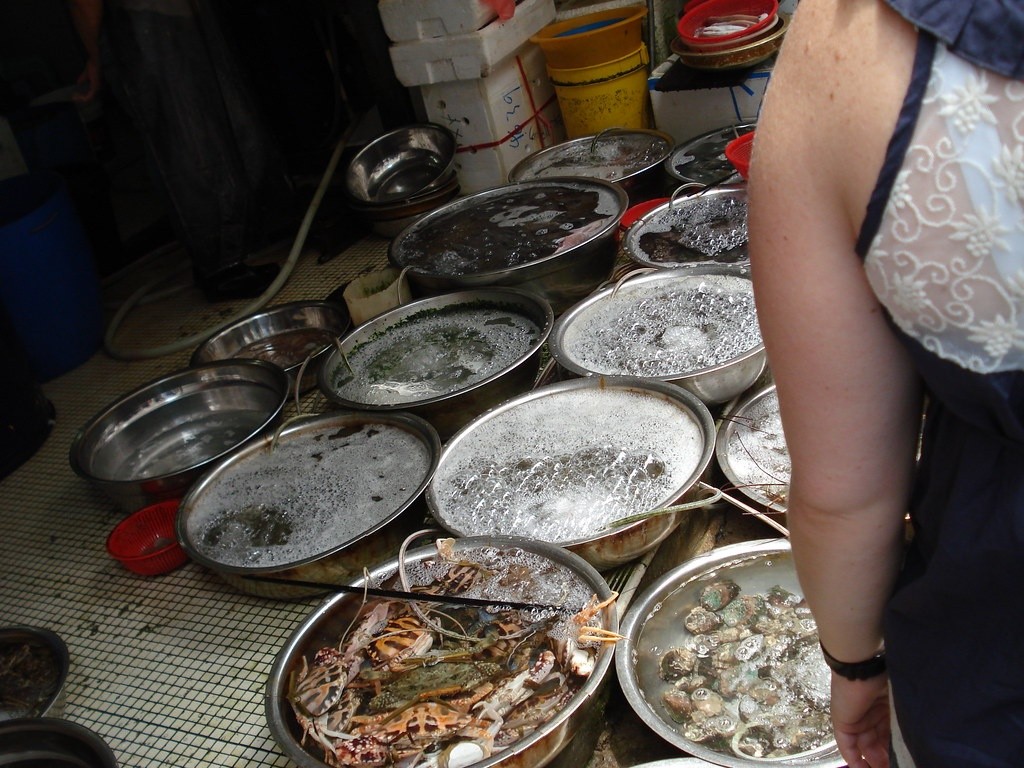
[747,0,1024,768]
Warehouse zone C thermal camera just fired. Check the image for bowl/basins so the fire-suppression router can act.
[530,5,648,68]
[671,0,787,69]
[0,122,913,768]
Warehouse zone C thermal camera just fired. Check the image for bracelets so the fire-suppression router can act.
[816,637,888,681]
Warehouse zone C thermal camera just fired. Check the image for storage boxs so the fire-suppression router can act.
[649,54,776,148]
[378,0,519,43]
[420,41,567,197]
[388,0,557,87]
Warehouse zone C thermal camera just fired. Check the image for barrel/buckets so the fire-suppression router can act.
[547,40,650,140]
[0,302,58,479]
[0,172,104,382]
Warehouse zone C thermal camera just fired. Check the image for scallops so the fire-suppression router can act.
[659,578,831,760]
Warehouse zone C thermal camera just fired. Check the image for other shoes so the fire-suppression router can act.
[249,185,337,244]
[200,260,280,304]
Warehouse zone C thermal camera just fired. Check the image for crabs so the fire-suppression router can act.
[283,539,626,768]
